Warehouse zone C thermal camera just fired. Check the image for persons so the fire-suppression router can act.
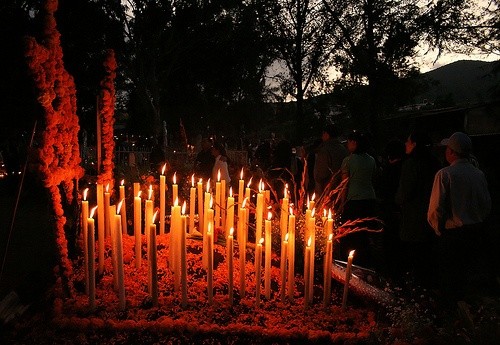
[1,125,442,296]
[426,132,492,325]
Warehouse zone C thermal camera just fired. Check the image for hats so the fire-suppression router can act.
[441,132,471,154]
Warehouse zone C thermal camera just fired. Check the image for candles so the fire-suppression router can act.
[78,158,356,317]
